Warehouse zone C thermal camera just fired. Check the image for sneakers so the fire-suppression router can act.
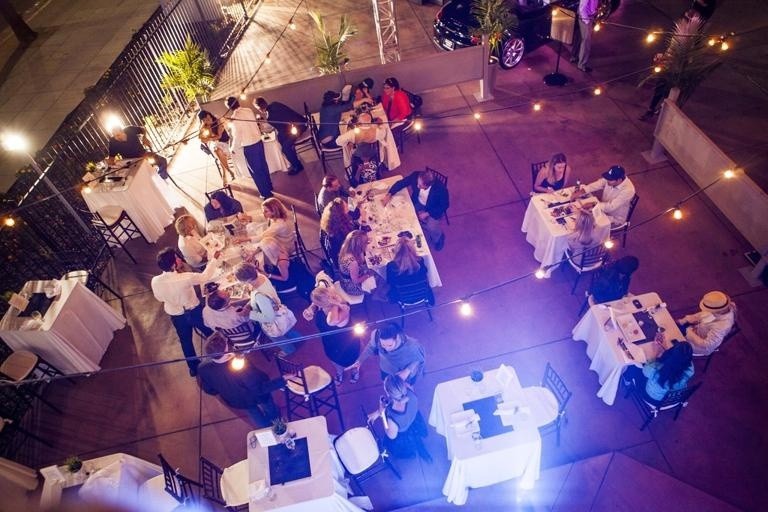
[349,371,360,383]
[334,370,344,386]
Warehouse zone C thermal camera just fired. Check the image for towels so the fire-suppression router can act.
[493,406,527,417]
[622,343,647,366]
[449,413,481,428]
[44,278,62,298]
[38,479,66,510]
[248,481,268,501]
[19,319,42,331]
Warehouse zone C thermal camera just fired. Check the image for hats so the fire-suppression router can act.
[699,291,731,312]
[359,78,374,88]
[602,165,625,180]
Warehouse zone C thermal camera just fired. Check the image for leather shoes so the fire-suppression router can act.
[434,233,445,251]
[288,165,304,175]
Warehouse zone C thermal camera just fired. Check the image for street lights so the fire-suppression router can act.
[4,133,93,235]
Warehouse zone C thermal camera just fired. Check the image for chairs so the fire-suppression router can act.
[427,167,451,226]
[193,457,248,512]
[531,159,550,194]
[201,141,233,180]
[59,251,122,300]
[530,160,547,190]
[293,117,322,156]
[619,364,701,433]
[272,352,345,433]
[140,143,180,189]
[612,195,640,246]
[692,321,739,371]
[525,362,571,447]
[290,205,313,272]
[74,204,149,265]
[335,405,402,497]
[344,161,356,187]
[311,129,345,174]
[312,193,322,215]
[158,454,201,504]
[394,115,422,152]
[563,241,608,292]
[0,351,76,415]
[392,279,432,329]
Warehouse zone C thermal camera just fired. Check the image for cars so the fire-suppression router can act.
[431,0,621,70]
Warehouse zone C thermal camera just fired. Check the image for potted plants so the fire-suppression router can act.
[270,417,294,444]
[64,457,90,478]
[85,162,104,175]
[2,291,30,312]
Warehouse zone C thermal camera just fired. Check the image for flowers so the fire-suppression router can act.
[470,0,518,61]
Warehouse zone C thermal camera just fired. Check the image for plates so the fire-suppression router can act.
[200,232,223,253]
[544,202,578,220]
[616,313,646,343]
[230,284,245,299]
[375,229,416,249]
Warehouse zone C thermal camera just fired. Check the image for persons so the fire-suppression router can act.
[175,215,209,271]
[577,0,597,73]
[245,238,300,298]
[621,341,694,408]
[254,96,308,175]
[568,209,609,264]
[301,288,359,386]
[196,331,282,428]
[534,153,571,193]
[343,322,424,390]
[335,113,386,144]
[316,86,357,148]
[316,174,351,214]
[587,255,639,306]
[349,142,389,188]
[103,124,168,182]
[379,169,449,251]
[674,291,734,357]
[196,291,254,349]
[149,248,220,377]
[379,76,413,149]
[227,94,273,202]
[197,110,234,187]
[352,78,374,108]
[232,263,301,358]
[315,198,368,262]
[204,191,244,221]
[337,230,389,303]
[383,236,435,305]
[571,165,636,227]
[232,198,297,255]
[366,374,428,478]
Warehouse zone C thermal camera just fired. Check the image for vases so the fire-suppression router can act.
[481,57,498,99]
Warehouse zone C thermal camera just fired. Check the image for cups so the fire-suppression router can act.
[32,311,44,323]
